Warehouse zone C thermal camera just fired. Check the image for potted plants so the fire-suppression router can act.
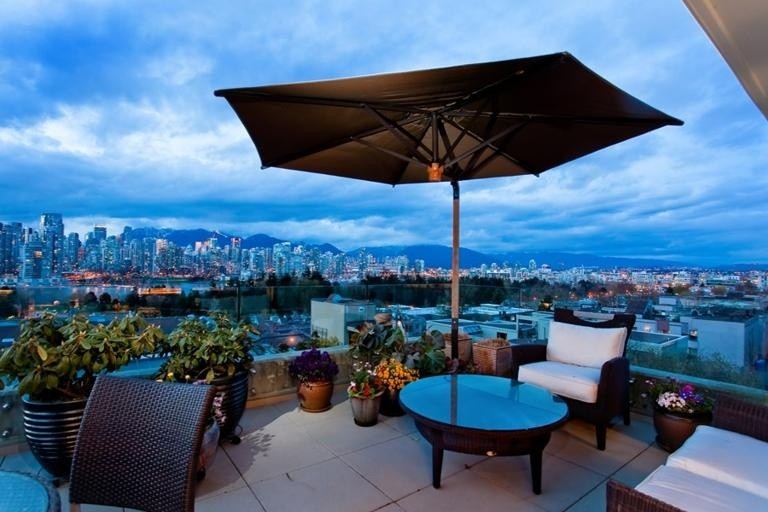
[0,308,158,483]
[155,314,260,445]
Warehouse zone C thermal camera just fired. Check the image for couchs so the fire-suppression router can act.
[607,392,768,512]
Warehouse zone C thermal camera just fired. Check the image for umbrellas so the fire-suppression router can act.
[213,50,685,362]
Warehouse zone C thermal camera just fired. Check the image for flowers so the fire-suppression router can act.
[348,360,384,397]
[374,358,420,400]
[156,371,225,426]
[643,377,715,411]
[289,345,339,381]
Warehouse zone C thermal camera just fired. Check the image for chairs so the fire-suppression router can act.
[513,308,635,451]
[69,376,217,512]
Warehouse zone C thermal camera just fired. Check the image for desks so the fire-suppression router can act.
[0,470,62,512]
[399,373,570,495]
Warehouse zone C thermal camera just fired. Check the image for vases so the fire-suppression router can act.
[381,387,406,416]
[347,385,385,426]
[195,417,221,481]
[652,406,712,453]
[296,376,334,412]
